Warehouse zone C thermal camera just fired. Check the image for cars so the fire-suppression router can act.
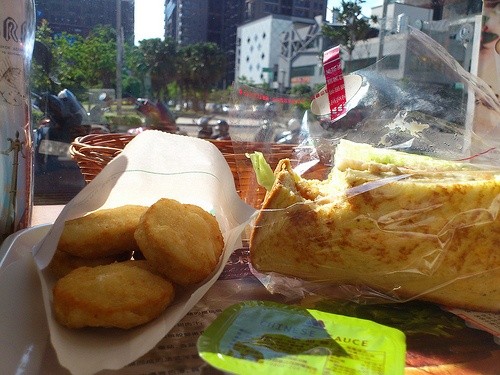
[136,95,281,119]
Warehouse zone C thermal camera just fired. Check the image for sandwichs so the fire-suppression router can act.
[245,138,500,314]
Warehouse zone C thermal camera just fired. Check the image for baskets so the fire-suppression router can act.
[69,131,330,209]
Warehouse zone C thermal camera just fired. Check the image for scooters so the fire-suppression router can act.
[30,39,96,206]
[192,114,231,142]
[251,114,283,143]
[87,91,111,133]
